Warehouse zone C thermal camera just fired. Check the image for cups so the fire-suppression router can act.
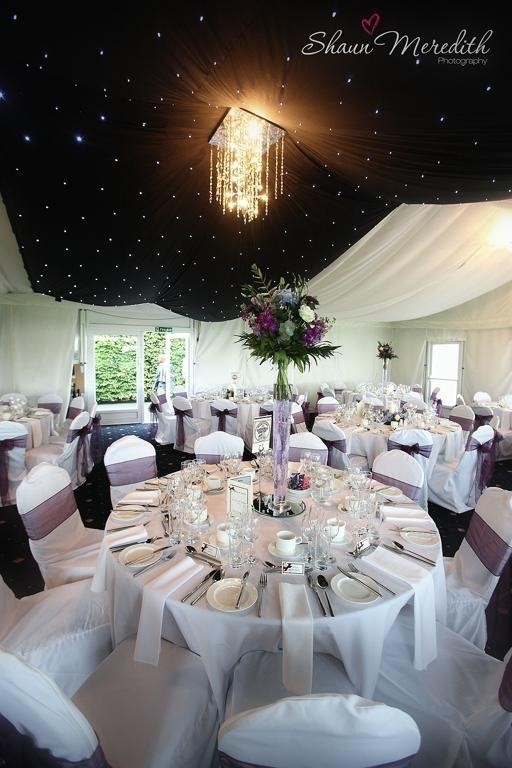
[319,404,457,440]
[158,448,382,582]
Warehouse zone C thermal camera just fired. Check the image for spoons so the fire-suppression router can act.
[305,573,333,620]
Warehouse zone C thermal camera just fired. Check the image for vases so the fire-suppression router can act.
[382,358,391,388]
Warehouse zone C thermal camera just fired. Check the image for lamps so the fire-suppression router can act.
[207,107,287,225]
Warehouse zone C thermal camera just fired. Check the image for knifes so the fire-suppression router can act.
[234,569,251,607]
[181,566,224,607]
[380,540,437,568]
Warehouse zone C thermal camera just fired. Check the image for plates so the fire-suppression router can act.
[197,390,264,405]
[400,524,440,546]
[331,573,380,606]
[114,474,166,571]
[206,576,257,612]
[374,482,406,498]
[2,408,49,424]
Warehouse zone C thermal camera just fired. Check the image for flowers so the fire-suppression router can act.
[378,341,400,360]
[232,262,342,402]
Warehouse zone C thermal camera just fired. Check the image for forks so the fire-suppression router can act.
[347,562,398,597]
[255,573,268,620]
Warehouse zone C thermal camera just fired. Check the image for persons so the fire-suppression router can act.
[150,353,167,413]
